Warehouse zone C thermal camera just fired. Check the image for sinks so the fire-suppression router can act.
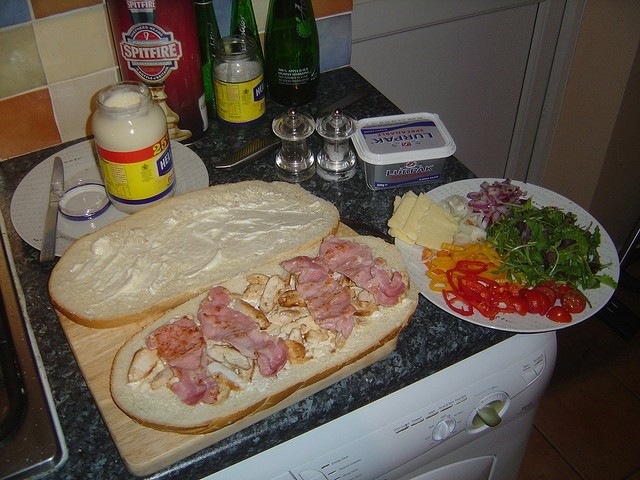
[0,231,63,480]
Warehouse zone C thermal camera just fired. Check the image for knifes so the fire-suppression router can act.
[40,157,63,267]
[215,94,357,170]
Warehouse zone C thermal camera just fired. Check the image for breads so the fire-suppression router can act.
[47,179,341,330]
[110,234,420,435]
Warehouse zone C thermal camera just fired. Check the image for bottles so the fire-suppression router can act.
[315,106,360,183]
[196,0,227,121]
[212,36,267,128]
[271,108,316,182]
[208,1,267,66]
[91,80,176,215]
[264,0,320,109]
[105,0,210,144]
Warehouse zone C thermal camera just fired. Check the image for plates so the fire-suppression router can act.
[394,176,621,333]
[9,136,210,262]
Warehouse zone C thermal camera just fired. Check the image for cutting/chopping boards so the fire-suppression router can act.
[55,220,400,476]
